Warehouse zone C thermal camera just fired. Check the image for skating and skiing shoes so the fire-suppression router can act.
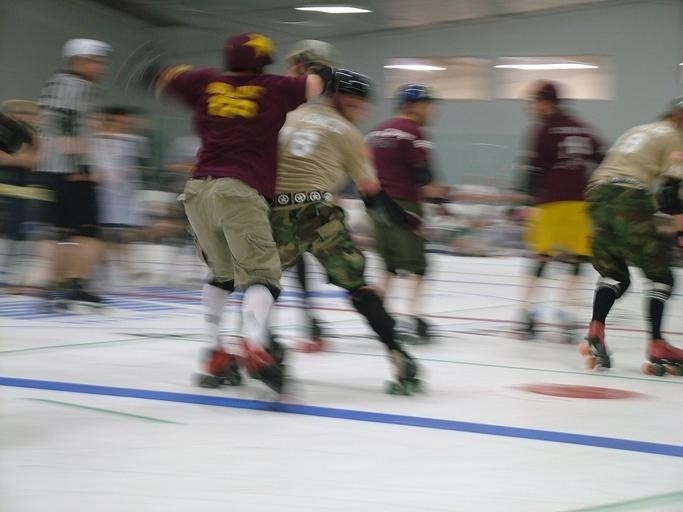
[643,336,683,379]
[578,334,613,374]
[193,333,278,397]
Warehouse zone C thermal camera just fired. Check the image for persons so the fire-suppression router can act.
[505,78,607,345]
[583,94,683,369]
[1,36,452,393]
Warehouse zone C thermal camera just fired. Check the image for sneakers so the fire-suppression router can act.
[384,313,427,381]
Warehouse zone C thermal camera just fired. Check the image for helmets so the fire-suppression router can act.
[60,37,112,59]
[224,32,273,70]
[332,69,373,99]
[391,83,437,104]
